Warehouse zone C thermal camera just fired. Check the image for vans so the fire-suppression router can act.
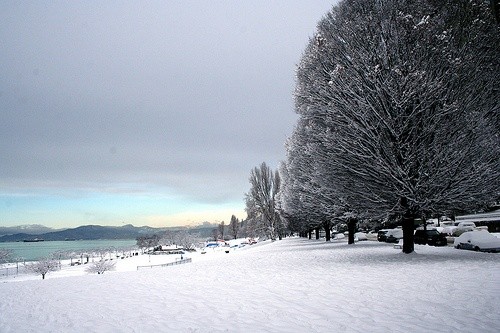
[414,229,447,247]
[378,230,400,244]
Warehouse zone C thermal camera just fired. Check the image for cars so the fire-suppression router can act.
[354,233,367,241]
[453,230,500,253]
